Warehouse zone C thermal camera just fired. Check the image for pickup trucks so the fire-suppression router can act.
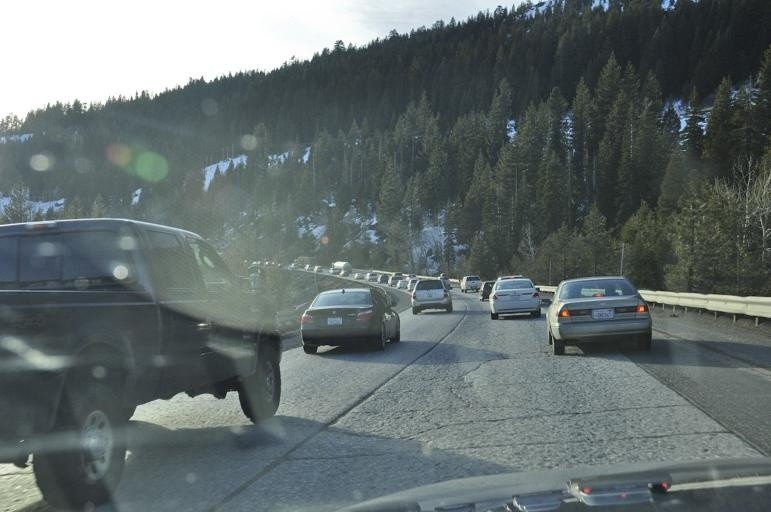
[2,219,283,511]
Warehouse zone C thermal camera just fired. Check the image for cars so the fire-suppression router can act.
[459,275,482,293]
[245,260,452,293]
[478,281,496,301]
[411,279,454,314]
[490,277,542,321]
[544,275,652,358]
[298,287,400,354]
[321,454,771,512]
